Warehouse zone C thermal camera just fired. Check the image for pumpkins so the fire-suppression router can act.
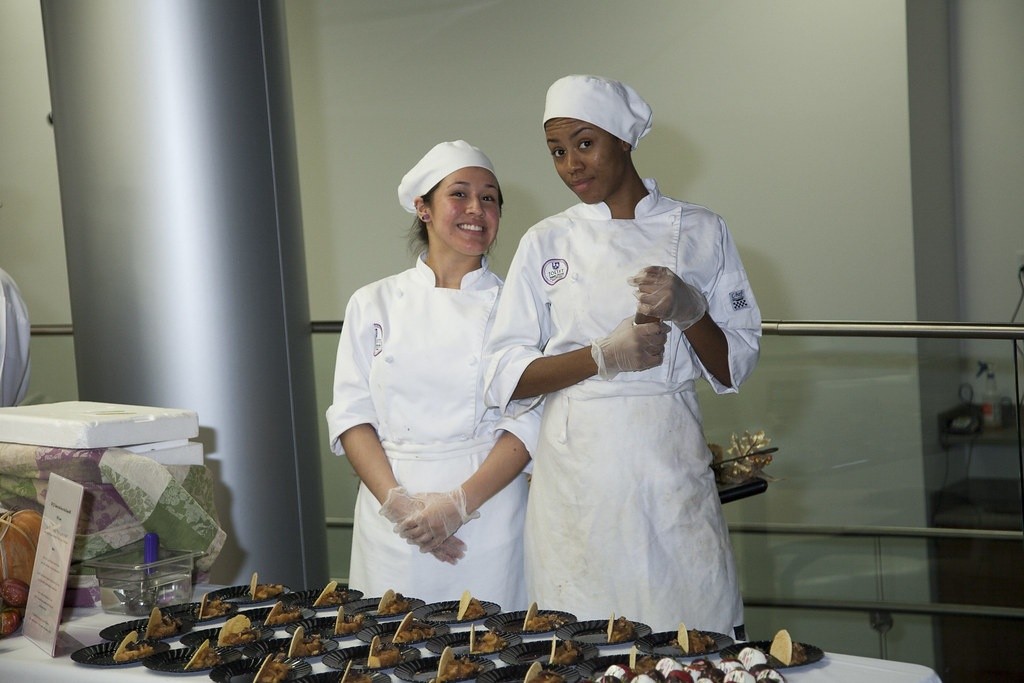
[0,499,43,617]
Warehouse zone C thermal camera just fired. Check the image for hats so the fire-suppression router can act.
[396,140,499,213]
[542,74,654,150]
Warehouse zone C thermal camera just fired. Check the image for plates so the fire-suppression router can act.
[71,583,825,683]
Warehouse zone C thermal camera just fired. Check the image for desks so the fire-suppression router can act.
[0,582,942,683]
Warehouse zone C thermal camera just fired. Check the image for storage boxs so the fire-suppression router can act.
[0,401,228,608]
[83,545,207,616]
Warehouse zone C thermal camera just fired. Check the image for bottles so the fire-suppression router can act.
[633,312,660,325]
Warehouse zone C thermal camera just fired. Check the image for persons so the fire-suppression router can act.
[483,74,765,640]
[327,140,533,612]
